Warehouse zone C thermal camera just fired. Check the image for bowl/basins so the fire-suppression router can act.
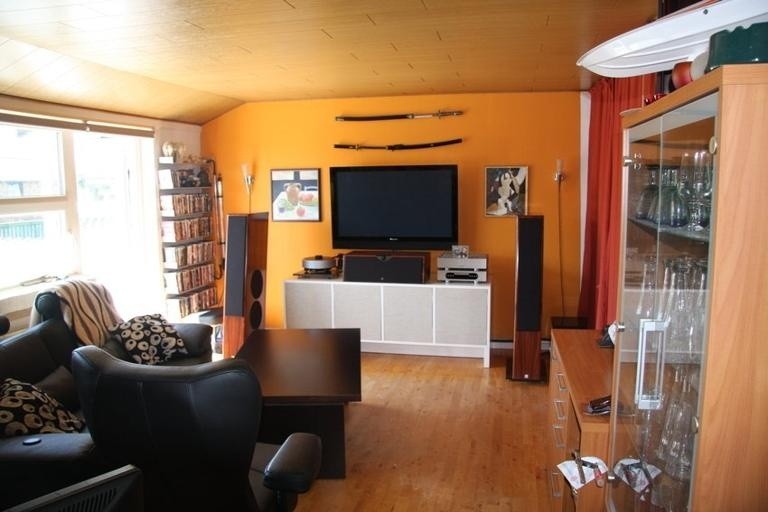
[644,93,666,105]
[671,61,693,89]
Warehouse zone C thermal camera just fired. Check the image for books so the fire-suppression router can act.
[158,168,219,320]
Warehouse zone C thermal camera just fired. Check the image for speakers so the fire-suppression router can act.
[512,215,544,380]
[223,213,269,360]
[342,252,431,283]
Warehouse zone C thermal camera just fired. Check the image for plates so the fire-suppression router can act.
[619,107,641,117]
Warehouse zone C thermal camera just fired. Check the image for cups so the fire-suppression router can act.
[631,148,714,512]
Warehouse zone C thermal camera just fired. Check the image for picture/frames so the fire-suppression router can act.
[483,165,530,219]
[269,167,323,223]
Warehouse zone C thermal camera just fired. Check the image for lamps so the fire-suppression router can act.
[552,159,568,317]
[242,163,258,215]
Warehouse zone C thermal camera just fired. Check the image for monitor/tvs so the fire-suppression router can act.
[328,163,458,251]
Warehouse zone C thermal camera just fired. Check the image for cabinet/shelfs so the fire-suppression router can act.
[608,64,768,511]
[286,282,489,346]
[158,162,219,322]
[545,328,615,510]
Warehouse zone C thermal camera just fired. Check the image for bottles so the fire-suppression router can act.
[197,166,209,187]
